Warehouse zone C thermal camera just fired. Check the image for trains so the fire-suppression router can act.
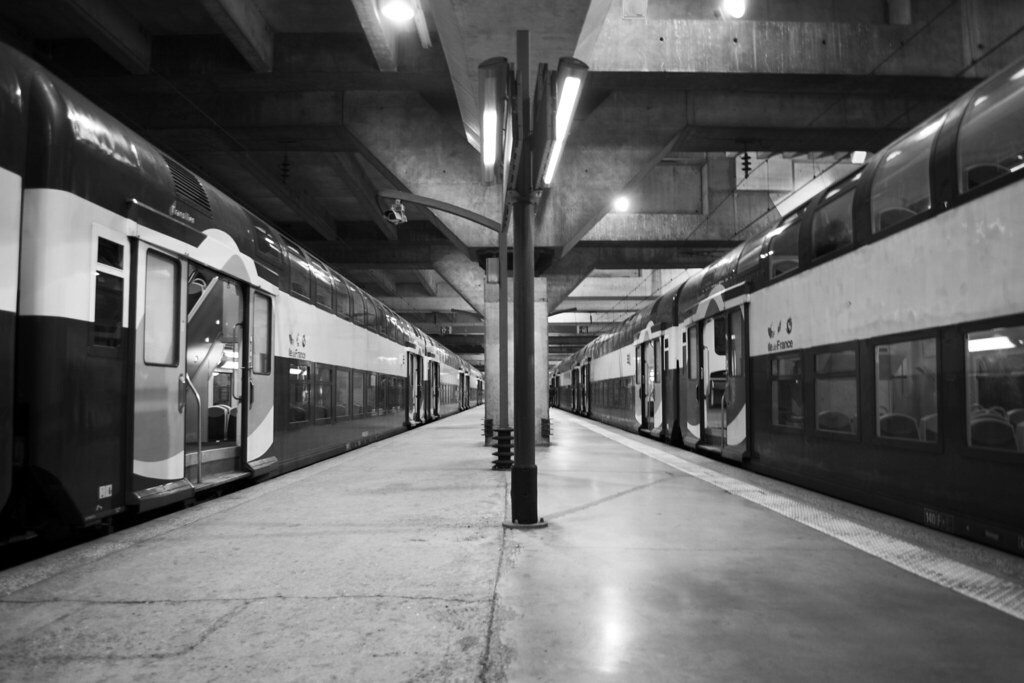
[0,39,485,562]
[549,52,1024,554]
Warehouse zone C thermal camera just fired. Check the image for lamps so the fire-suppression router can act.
[534,56,590,189]
[479,56,508,187]
